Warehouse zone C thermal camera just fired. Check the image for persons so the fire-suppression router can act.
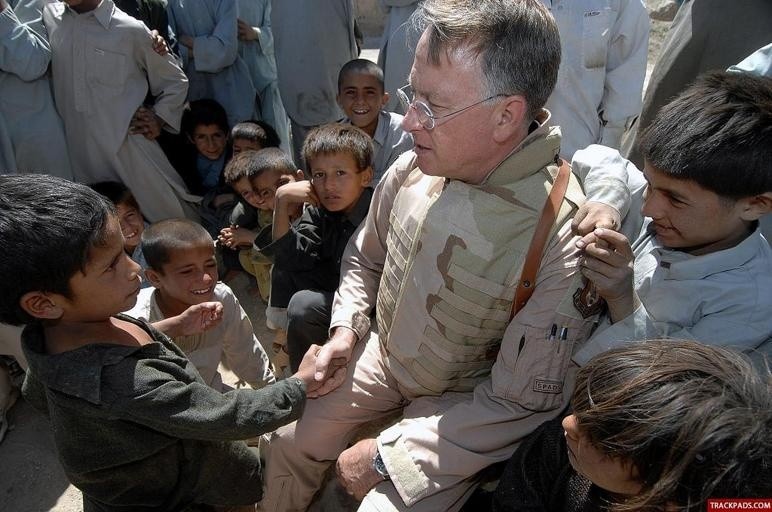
[0,1,771,511]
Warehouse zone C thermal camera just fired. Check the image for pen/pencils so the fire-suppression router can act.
[549,321,569,355]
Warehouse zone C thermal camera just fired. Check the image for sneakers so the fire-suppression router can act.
[0,386,19,446]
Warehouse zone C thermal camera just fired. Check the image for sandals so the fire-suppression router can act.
[269,345,290,382]
[272,327,288,356]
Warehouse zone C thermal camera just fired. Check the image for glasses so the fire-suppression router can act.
[395,82,510,131]
